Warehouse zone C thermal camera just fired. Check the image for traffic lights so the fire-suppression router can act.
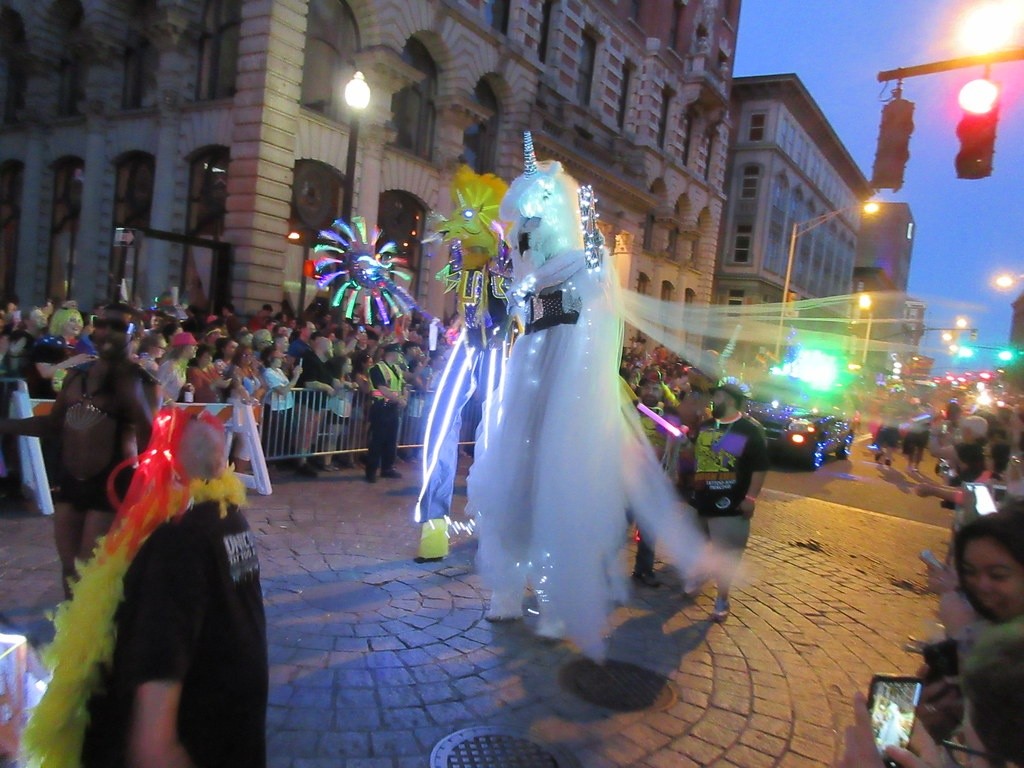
[953,78,1001,179]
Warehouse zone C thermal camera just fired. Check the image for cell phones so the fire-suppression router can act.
[965,482,997,515]
[299,358,303,367]
[921,550,943,570]
[865,674,923,768]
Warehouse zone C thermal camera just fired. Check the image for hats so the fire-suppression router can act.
[172,333,198,347]
[641,369,661,384]
[269,350,287,358]
[709,384,744,401]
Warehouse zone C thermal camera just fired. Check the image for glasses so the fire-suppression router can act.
[941,729,1006,768]
[242,354,252,357]
[263,340,274,344]
[158,346,169,351]
[92,316,136,333]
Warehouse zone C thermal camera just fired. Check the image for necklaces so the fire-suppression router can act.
[19,462,247,768]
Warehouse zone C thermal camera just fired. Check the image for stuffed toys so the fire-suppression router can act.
[412,131,748,665]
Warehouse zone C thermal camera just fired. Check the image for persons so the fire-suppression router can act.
[617,327,1024,768]
[0,310,464,768]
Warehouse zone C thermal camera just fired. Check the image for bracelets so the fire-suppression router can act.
[745,495,759,504]
[8,354,21,358]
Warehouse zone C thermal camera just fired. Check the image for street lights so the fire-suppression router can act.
[860,293,968,386]
[771,198,879,390]
[333,70,366,411]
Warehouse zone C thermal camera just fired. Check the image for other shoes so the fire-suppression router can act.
[299,463,317,478]
[632,565,660,587]
[714,600,730,620]
[485,608,523,621]
[366,470,376,482]
[537,623,567,639]
[322,464,339,471]
[683,581,699,594]
[886,460,891,465]
[381,470,401,478]
[875,453,880,460]
[315,460,324,469]
[908,466,919,471]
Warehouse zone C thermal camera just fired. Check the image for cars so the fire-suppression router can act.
[746,383,857,470]
